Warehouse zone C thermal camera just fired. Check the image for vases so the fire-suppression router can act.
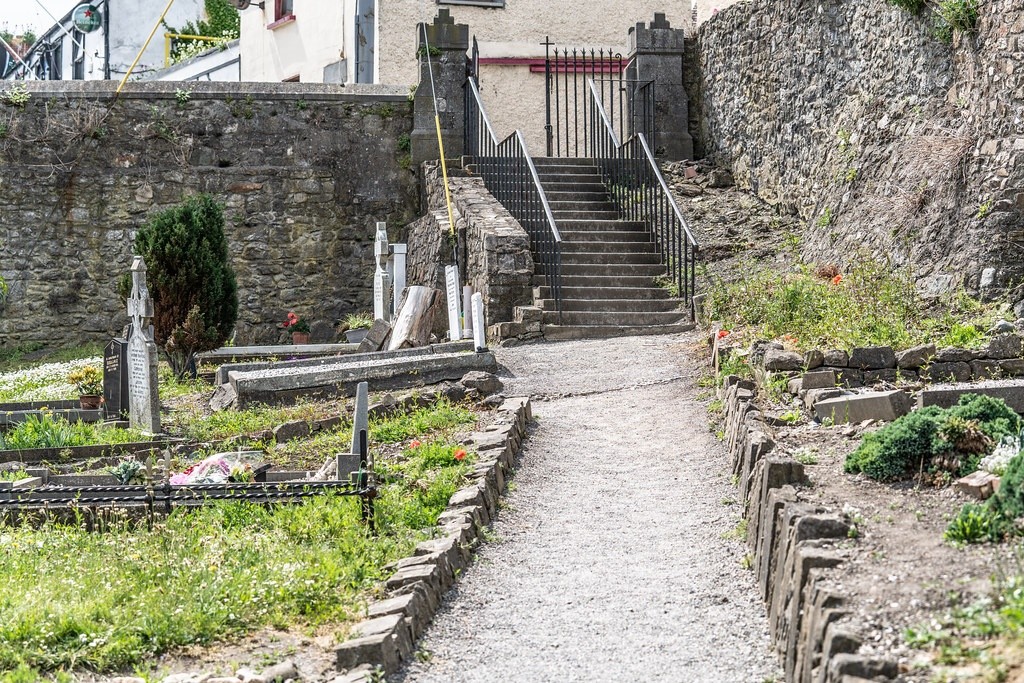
[293,331,309,345]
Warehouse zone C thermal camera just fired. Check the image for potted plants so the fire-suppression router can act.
[337,311,376,343]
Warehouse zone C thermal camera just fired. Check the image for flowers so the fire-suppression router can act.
[283,313,311,335]
